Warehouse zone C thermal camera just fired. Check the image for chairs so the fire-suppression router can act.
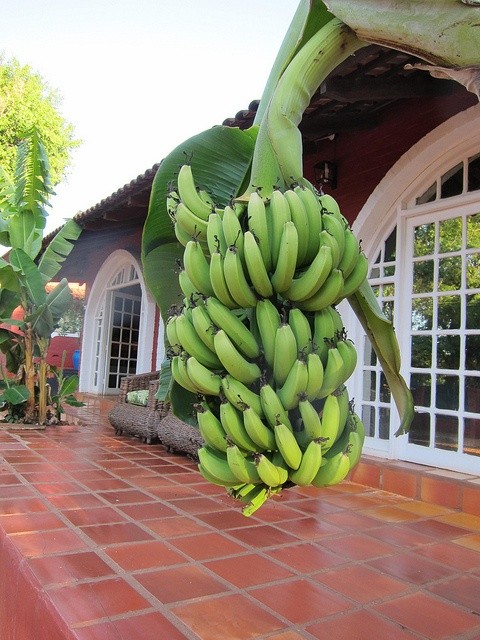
[110,368,166,443]
[156,380,206,461]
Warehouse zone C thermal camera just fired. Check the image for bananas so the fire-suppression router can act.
[163,158,368,519]
